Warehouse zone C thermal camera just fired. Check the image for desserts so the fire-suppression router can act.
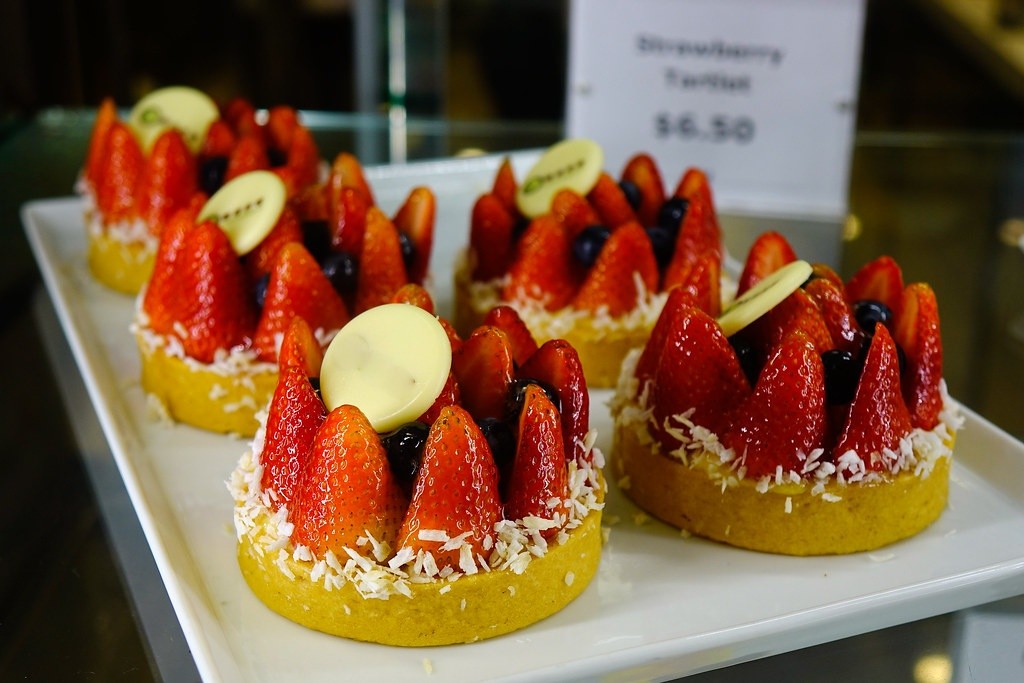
[78,87,965,650]
